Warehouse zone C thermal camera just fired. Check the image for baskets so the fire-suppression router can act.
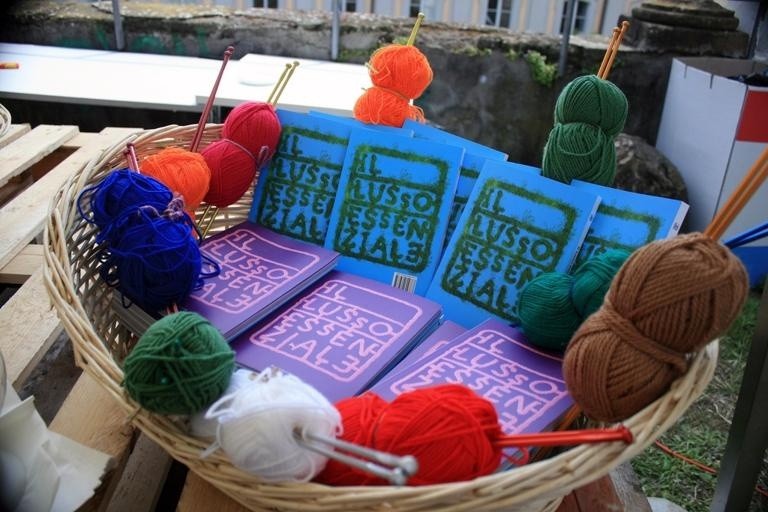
[40,114,728,512]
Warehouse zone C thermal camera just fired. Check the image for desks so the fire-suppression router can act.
[195,53,414,125]
[0,41,238,126]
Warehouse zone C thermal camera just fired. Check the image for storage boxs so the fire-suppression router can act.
[655,56,768,290]
